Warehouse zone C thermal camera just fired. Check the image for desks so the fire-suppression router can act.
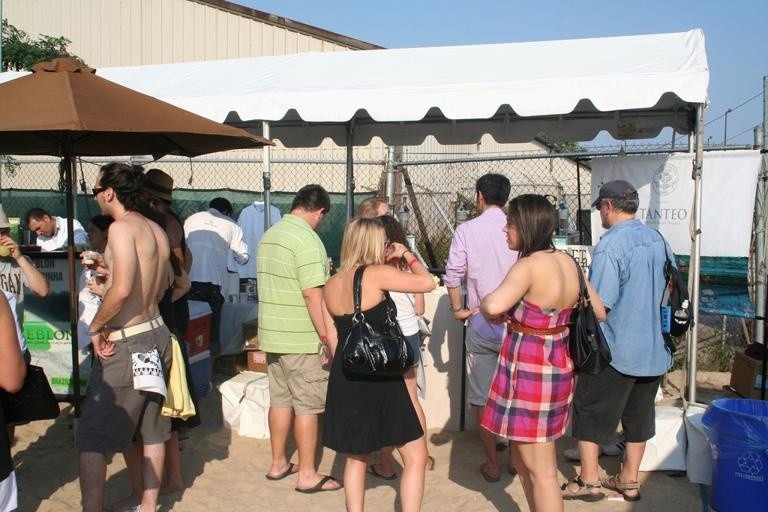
[220,299,259,378]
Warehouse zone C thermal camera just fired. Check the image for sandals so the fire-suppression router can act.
[562,477,606,502]
[605,474,641,500]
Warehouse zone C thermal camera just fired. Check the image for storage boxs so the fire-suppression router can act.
[187,350,211,398]
[730,349,768,401]
[247,351,267,372]
[241,321,267,351]
[185,299,213,356]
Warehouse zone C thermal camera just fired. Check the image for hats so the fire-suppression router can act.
[591,181,637,206]
[142,169,174,203]
[0,205,13,229]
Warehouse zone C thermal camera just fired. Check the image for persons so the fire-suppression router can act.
[479,192,607,512]
[182,197,250,393]
[68,214,114,418]
[0,290,30,512]
[87,209,192,512]
[444,173,523,483]
[0,204,49,444]
[357,196,430,369]
[144,167,201,451]
[366,214,436,481]
[24,207,90,253]
[556,179,679,502]
[255,183,345,494]
[75,162,176,512]
[320,217,437,512]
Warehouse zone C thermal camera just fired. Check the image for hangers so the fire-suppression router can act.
[254,191,265,206]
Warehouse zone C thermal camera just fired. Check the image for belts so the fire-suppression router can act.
[509,318,567,337]
[106,315,166,344]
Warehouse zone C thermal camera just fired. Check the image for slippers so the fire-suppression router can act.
[367,463,400,482]
[481,461,498,483]
[426,456,436,472]
[267,464,298,481]
[295,477,342,495]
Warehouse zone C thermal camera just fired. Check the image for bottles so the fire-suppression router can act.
[557,200,568,236]
[455,201,467,227]
[398,197,410,231]
[673,299,690,324]
[247,279,257,303]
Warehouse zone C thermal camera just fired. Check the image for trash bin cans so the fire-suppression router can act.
[703,399,767,512]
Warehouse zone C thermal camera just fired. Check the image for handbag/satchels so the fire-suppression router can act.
[651,225,694,353]
[340,263,415,381]
[0,367,62,421]
[566,252,612,377]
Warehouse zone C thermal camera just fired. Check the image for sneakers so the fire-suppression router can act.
[605,440,630,456]
[562,447,579,463]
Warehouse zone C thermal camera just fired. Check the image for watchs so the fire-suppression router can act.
[448,305,463,312]
[86,328,104,337]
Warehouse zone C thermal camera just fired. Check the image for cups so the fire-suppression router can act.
[83,248,96,265]
[0,235,10,257]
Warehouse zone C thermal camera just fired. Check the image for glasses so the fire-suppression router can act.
[91,188,109,196]
